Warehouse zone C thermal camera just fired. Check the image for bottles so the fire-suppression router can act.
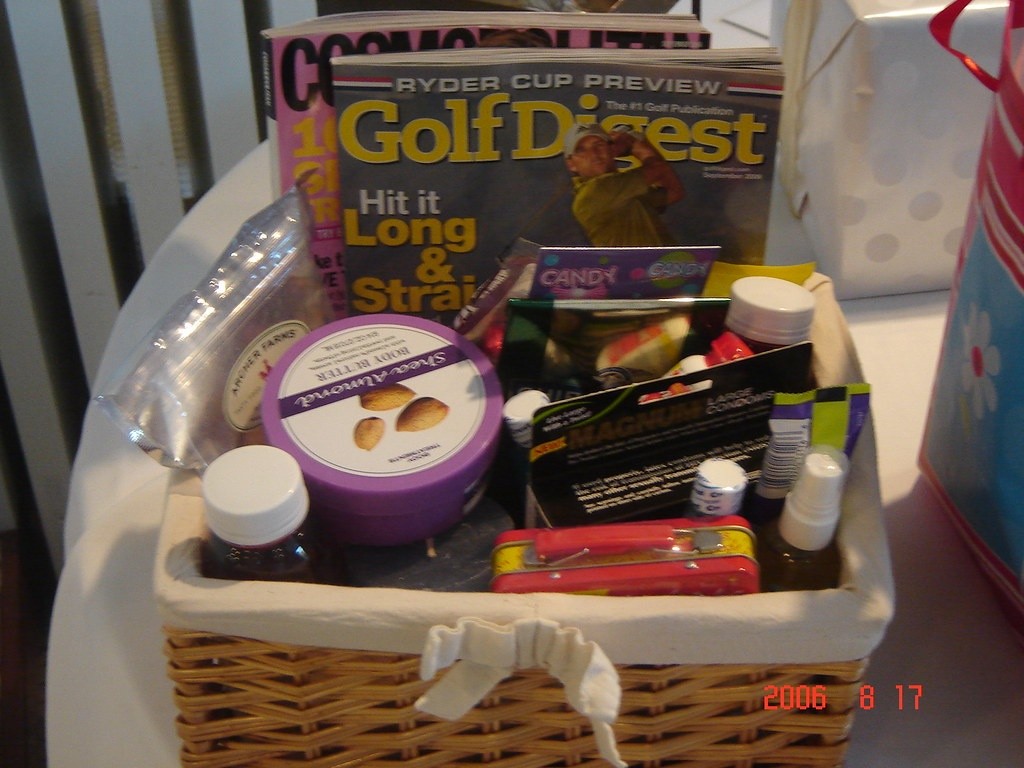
[198,445,345,585]
[637,276,841,595]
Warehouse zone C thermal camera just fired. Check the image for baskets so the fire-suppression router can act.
[152,270,871,768]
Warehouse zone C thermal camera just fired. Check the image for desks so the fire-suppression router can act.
[44,135,1024,768]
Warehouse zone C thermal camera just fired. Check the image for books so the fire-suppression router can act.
[329,48,787,328]
[261,10,714,320]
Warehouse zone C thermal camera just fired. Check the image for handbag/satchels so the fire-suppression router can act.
[917,0,1024,607]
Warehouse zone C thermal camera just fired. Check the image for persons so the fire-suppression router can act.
[562,123,684,248]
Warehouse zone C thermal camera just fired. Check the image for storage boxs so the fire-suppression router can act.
[522,338,817,527]
[489,515,759,597]
[768,0,1024,303]
